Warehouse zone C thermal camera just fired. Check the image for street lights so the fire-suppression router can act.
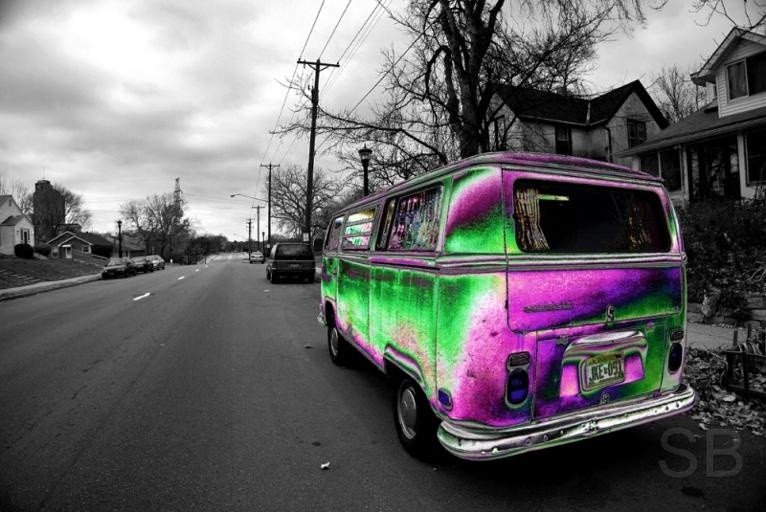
[359,143,372,197]
[262,232,265,256]
[230,194,270,257]
[118,220,122,258]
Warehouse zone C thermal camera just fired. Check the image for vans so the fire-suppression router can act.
[316,149,696,462]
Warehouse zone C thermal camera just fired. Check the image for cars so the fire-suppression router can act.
[250,252,265,264]
[101,254,165,280]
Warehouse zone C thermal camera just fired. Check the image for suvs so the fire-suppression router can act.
[265,243,315,282]
